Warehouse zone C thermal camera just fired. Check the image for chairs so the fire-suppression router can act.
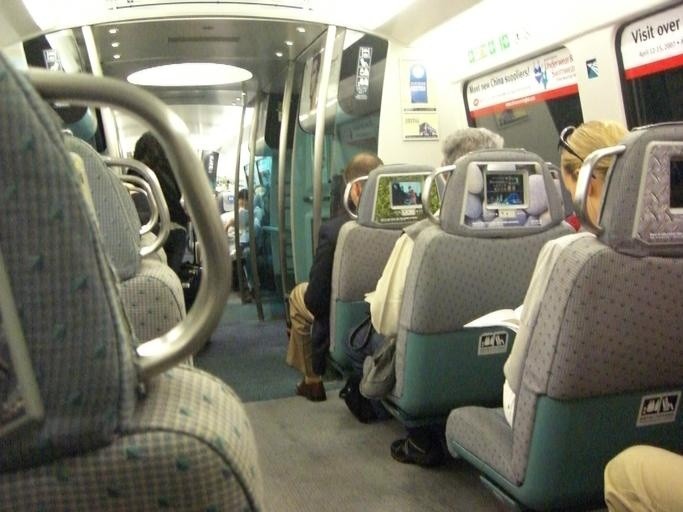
[0,50,265,511]
[325,120,683,512]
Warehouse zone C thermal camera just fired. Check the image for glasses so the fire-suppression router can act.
[558,125,596,179]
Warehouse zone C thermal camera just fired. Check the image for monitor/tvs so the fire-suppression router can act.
[389,181,425,210]
[483,169,530,209]
[670,158,683,214]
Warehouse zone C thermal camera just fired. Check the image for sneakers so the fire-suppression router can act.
[295,378,327,401]
[390,437,449,467]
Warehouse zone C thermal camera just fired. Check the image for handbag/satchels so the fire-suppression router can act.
[339,376,376,423]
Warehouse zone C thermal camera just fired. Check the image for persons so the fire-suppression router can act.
[366,127,505,465]
[287,152,384,403]
[604,445,683,512]
[225,189,256,302]
[503,119,629,428]
[125,131,188,277]
